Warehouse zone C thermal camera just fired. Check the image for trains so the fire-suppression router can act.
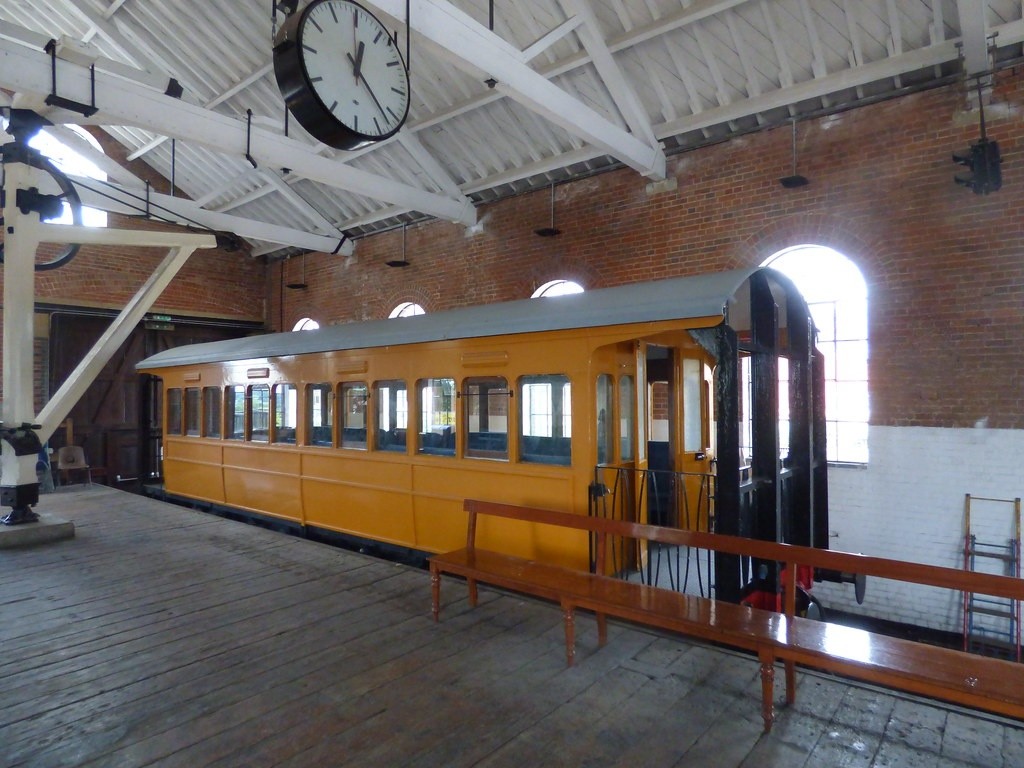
[131,265,828,617]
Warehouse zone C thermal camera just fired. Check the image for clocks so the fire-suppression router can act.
[271,1,411,150]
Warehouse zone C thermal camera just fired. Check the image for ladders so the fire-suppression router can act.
[967,533,1018,659]
[963,493,1022,657]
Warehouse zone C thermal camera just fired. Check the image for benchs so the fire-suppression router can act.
[424,498,1024,735]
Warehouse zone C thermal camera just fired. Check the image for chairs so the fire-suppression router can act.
[274,416,675,525]
